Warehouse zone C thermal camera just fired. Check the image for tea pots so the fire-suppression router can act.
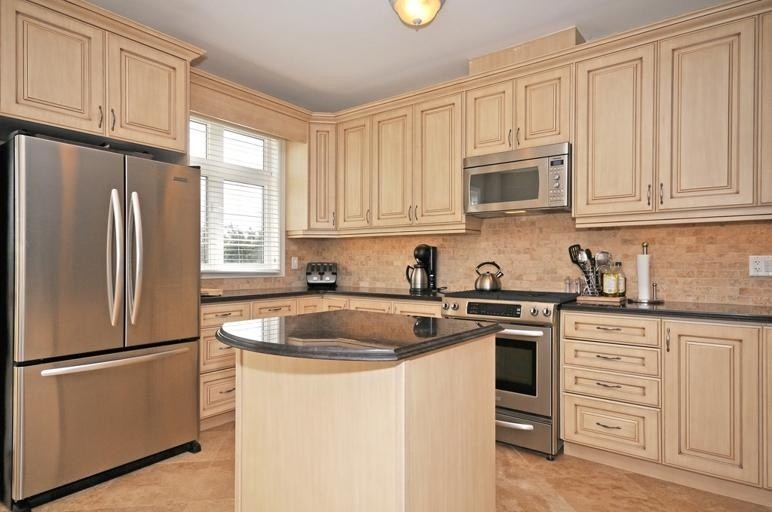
[474,261,504,290]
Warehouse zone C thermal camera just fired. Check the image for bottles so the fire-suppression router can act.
[612,261,627,297]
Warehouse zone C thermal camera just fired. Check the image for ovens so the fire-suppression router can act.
[494,324,564,460]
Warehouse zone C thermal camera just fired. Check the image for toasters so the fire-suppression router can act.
[305,261,338,286]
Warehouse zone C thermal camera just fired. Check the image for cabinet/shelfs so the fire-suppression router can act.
[283,120,335,240]
[1,0,190,163]
[201,296,444,430]
[460,64,570,159]
[371,93,481,236]
[658,315,771,509]
[570,7,772,231]
[335,114,371,238]
[558,308,662,480]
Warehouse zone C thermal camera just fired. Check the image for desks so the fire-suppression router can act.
[216,307,505,512]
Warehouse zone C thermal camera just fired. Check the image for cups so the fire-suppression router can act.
[602,272,619,298]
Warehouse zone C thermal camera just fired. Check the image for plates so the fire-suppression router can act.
[201,288,223,297]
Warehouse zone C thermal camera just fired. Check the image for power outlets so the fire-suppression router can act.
[749,255,772,277]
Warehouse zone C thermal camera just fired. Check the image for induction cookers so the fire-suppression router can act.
[440,290,579,324]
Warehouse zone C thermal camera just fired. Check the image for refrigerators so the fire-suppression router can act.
[1,129,204,510]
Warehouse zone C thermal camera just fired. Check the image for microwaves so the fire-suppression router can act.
[460,139,572,219]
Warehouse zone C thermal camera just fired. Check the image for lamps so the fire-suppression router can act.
[387,0,448,32]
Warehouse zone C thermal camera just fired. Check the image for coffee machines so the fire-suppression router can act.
[406,243,437,293]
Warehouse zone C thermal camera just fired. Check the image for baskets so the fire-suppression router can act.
[580,269,602,296]
